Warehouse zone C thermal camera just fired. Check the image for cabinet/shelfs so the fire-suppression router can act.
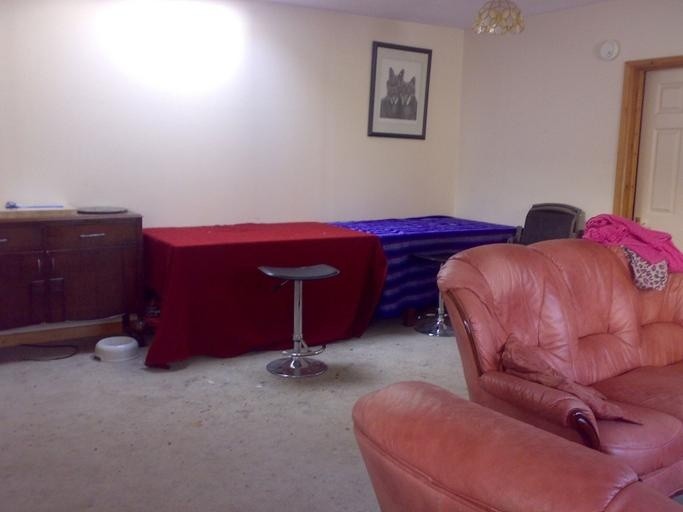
[0,208,147,348]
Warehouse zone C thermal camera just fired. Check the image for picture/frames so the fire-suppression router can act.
[367,40,433,141]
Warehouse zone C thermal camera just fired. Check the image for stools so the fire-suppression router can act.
[412,251,460,338]
[256,264,341,379]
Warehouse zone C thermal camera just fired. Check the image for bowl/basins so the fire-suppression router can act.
[94,337,138,362]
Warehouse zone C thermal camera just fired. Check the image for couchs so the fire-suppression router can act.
[437,237,681,496]
[353,380,681,512]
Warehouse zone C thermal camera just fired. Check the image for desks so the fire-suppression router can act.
[340,216,520,328]
[143,222,370,363]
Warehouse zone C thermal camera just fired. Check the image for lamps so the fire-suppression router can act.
[471,0,526,36]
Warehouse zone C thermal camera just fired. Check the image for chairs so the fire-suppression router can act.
[507,203,585,247]
